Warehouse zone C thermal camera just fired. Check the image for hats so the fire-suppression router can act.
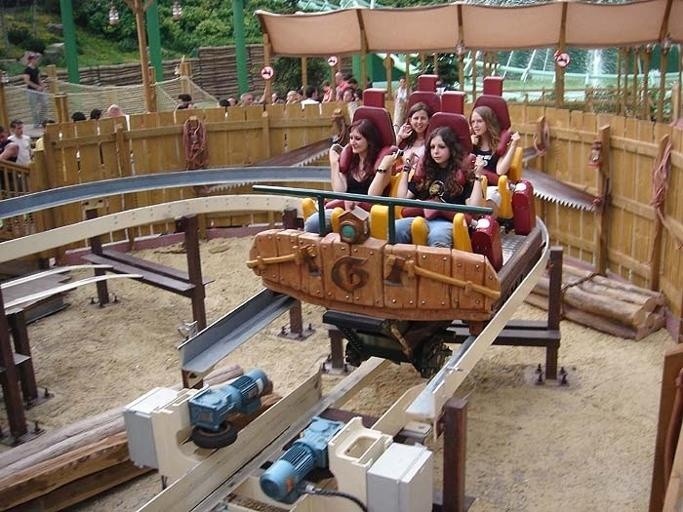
[27,53,40,59]
[105,104,124,117]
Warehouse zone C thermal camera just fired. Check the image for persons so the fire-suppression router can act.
[0,104,130,232]
[393,77,412,135]
[303,119,395,234]
[219,72,373,117]
[395,103,432,168]
[394,127,486,248]
[24,54,49,128]
[173,93,194,123]
[469,106,520,211]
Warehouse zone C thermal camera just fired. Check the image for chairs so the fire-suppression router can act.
[409,91,442,118]
[302,107,396,241]
[380,111,502,274]
[470,95,536,234]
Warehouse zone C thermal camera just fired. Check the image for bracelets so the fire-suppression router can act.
[404,166,411,170]
[471,175,483,182]
[403,169,410,173]
[376,168,387,174]
